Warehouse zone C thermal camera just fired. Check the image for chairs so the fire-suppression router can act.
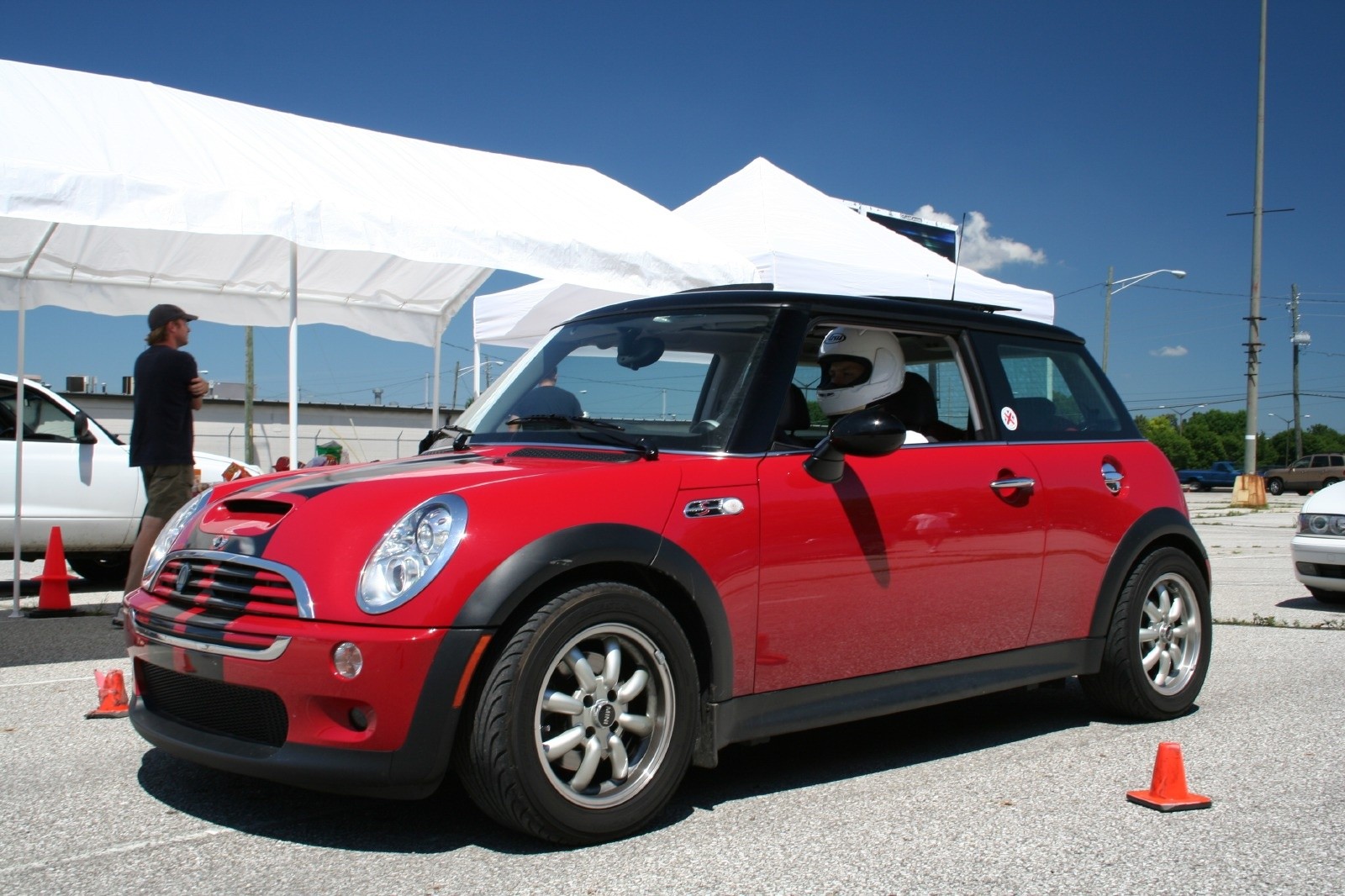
[828,372,940,458]
[759,382,812,457]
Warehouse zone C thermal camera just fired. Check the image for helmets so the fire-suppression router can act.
[816,327,904,413]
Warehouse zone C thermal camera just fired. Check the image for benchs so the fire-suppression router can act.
[961,395,1066,444]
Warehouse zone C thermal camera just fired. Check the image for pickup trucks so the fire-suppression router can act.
[1176,460,1264,493]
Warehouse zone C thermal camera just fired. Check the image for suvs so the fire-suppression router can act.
[1262,453,1345,496]
[114,279,1216,849]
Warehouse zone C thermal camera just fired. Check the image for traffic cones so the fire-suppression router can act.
[21,525,87,618]
[1124,739,1212,815]
[84,668,130,719]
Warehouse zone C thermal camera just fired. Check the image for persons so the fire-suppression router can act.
[113,305,208,626]
[505,364,586,432]
[816,325,929,445]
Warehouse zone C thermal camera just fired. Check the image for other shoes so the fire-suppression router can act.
[112,603,125,627]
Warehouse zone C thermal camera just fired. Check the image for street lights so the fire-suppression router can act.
[1100,264,1187,375]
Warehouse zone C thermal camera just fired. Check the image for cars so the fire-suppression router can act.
[0,372,267,587]
[1289,480,1345,607]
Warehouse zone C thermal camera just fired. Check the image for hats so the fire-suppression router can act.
[148,305,197,330]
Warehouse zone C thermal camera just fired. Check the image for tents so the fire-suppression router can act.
[0,56,1056,662]
